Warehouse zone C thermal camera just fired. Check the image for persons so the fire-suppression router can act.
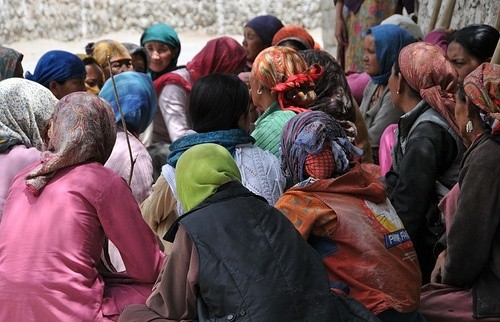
[0,92,168,322]
[333,0,418,77]
[274,111,423,322]
[342,15,500,322]
[0,15,375,273]
[117,143,337,322]
